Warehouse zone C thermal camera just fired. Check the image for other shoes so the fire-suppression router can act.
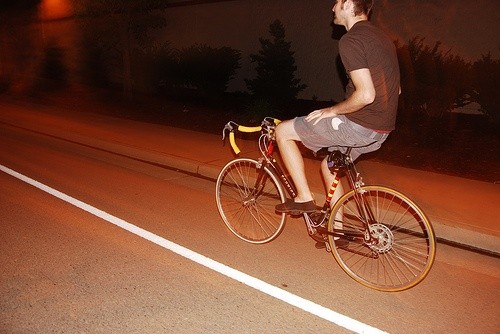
[275,199,318,214]
[315,237,350,251]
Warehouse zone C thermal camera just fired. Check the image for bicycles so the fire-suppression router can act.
[215,116,436,292]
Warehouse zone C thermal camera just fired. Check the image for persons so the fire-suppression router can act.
[272,0,402,251]
